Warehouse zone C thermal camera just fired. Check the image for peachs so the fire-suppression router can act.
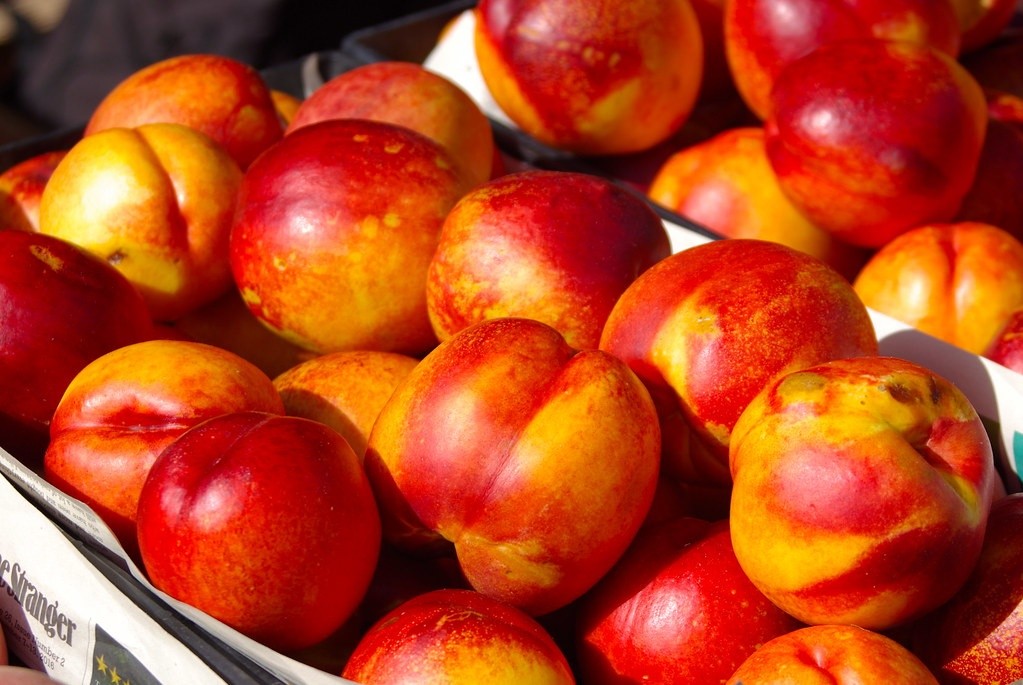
[0,0,1023,685]
